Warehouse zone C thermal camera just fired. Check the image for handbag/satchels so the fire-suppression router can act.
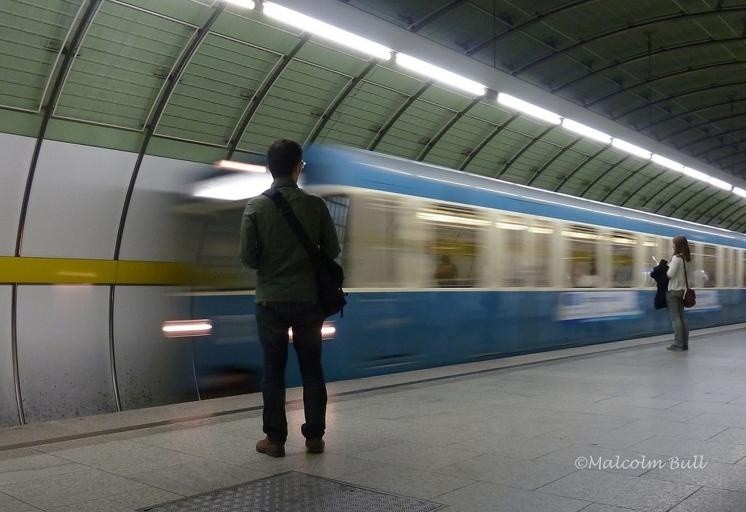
[310,248,349,319]
[683,288,697,308]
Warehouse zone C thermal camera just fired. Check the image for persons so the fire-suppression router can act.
[434,253,458,287]
[666,237,692,350]
[240,139,342,457]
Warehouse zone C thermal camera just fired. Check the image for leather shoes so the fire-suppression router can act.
[667,344,684,351]
[307,439,326,454]
[255,437,286,457]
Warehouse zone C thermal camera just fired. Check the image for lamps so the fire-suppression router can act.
[204,0,746,196]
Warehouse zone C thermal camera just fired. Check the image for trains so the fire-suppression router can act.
[168,141,746,403]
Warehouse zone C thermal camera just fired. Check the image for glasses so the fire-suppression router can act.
[298,158,307,170]
